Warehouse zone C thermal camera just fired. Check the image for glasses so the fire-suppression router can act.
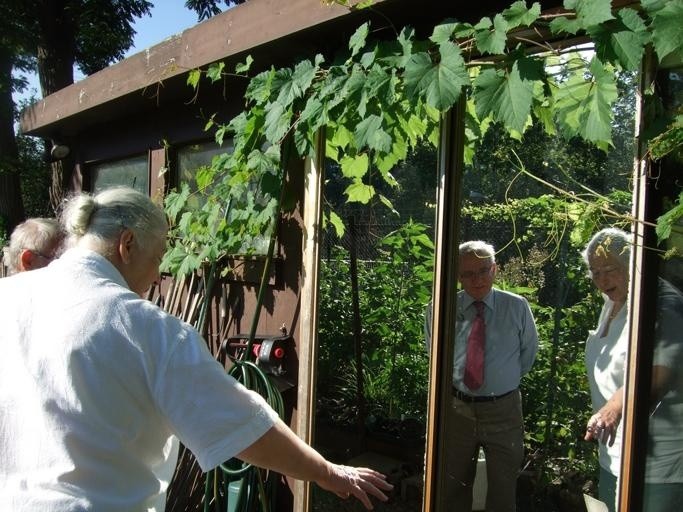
[457,265,494,279]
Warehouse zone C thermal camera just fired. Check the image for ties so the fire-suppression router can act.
[463,301,487,393]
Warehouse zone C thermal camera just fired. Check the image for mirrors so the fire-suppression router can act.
[425,30,653,512]
[637,60,682,512]
[294,125,450,511]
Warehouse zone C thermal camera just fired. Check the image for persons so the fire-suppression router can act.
[2,215,67,275]
[584,224,681,512]
[0,186,394,511]
[423,240,540,512]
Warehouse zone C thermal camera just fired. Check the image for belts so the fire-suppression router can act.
[450,386,519,404]
